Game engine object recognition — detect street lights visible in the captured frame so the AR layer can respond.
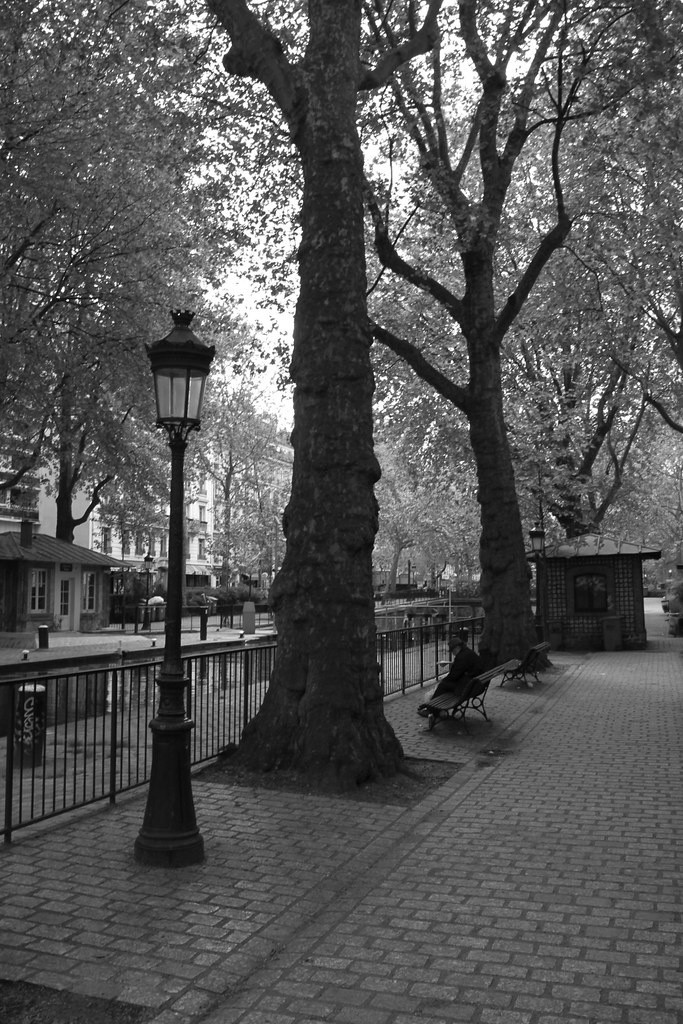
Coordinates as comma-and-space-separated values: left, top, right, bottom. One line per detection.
412, 562, 416, 584
142, 551, 154, 630
132, 304, 218, 870
528, 519, 544, 643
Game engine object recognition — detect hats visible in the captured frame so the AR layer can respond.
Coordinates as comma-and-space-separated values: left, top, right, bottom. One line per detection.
454, 626, 470, 642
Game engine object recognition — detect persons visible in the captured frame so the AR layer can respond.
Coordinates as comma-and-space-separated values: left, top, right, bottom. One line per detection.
416, 637, 482, 718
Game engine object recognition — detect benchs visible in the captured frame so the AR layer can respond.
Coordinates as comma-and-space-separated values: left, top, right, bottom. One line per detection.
495, 639, 553, 690
415, 656, 524, 737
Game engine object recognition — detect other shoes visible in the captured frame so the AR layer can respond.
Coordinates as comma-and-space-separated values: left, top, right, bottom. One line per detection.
419, 708, 431, 717
418, 702, 429, 710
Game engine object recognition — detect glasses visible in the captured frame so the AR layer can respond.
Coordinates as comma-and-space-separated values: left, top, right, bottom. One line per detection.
450, 635, 458, 639
